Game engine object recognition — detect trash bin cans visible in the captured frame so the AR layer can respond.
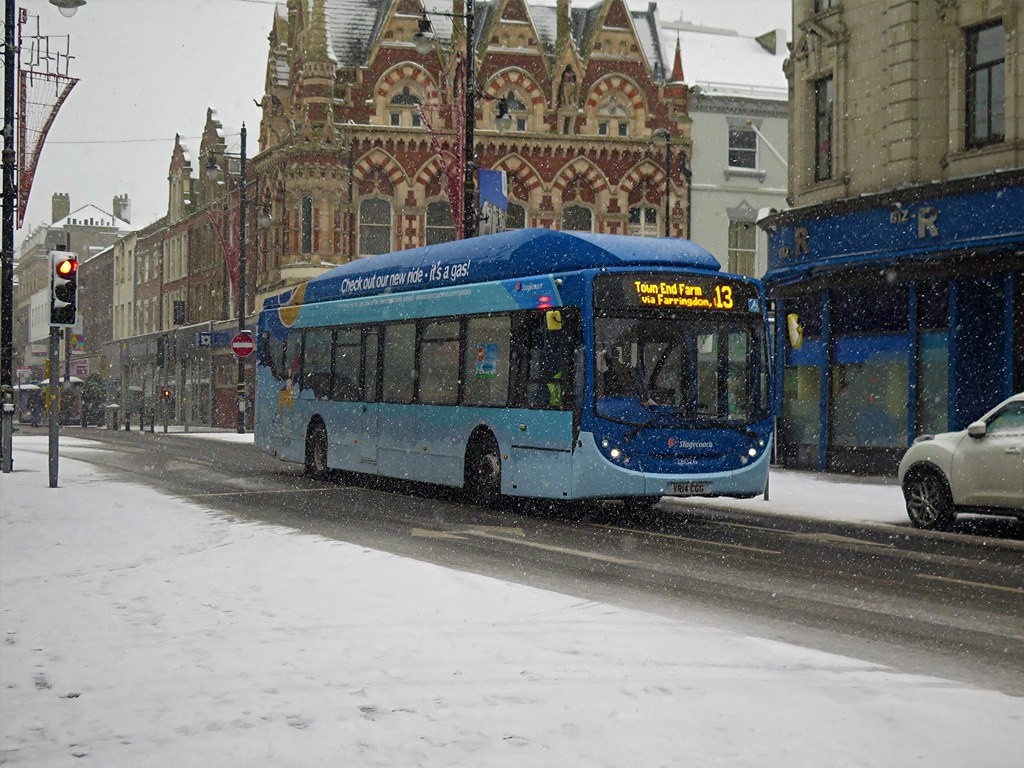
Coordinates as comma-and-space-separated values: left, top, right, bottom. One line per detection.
107, 404, 121, 430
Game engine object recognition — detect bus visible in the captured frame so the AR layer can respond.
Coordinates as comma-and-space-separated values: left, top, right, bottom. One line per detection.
253, 226, 805, 515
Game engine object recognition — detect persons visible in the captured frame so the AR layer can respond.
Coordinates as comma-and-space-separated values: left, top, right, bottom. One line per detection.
605, 346, 642, 397
29, 398, 41, 427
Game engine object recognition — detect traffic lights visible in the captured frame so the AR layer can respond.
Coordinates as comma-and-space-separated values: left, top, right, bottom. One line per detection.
48, 251, 79, 328
164, 391, 171, 396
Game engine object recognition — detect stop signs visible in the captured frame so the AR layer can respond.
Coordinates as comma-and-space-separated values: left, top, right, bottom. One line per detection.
231, 333, 254, 357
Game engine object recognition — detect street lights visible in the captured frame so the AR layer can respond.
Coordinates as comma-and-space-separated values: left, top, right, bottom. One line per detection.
205, 120, 247, 434
1, 0, 87, 470
414, 1, 513, 240
652, 128, 671, 237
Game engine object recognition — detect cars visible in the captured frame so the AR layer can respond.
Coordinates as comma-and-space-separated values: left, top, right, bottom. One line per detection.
897, 392, 1024, 532
